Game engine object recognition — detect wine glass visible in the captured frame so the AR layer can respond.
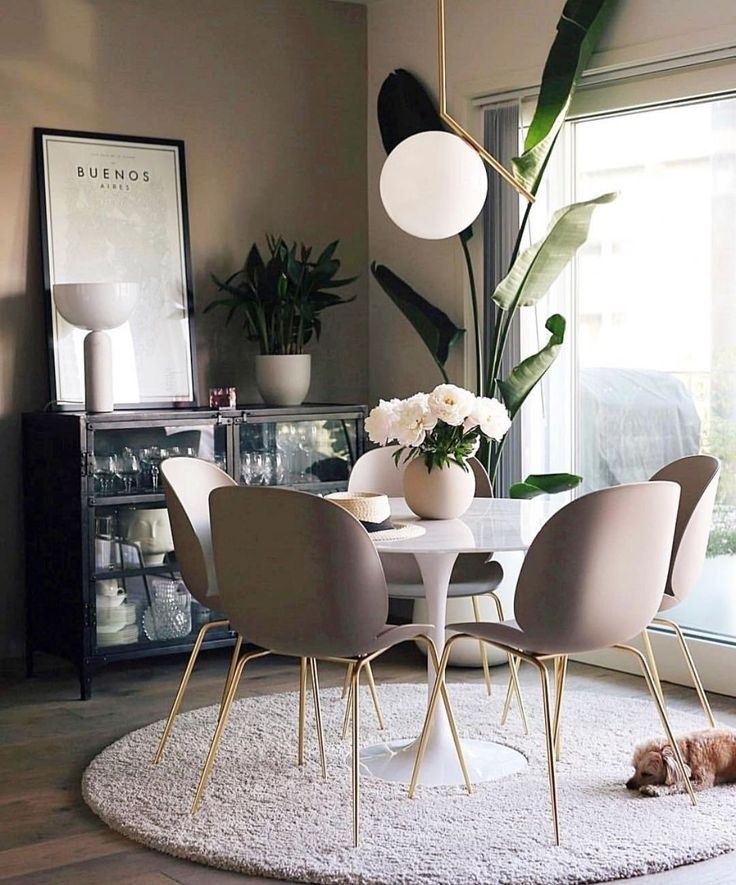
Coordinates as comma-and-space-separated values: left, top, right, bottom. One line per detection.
94, 446, 169, 496
276, 422, 322, 484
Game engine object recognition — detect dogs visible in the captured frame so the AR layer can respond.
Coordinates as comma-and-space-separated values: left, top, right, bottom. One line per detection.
625, 727, 736, 797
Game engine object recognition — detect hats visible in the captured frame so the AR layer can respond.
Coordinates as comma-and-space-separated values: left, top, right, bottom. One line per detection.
324, 491, 426, 541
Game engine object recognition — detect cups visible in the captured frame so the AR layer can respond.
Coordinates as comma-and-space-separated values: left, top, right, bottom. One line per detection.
96, 578, 125, 596
166, 447, 196, 457
241, 449, 290, 486
209, 387, 236, 410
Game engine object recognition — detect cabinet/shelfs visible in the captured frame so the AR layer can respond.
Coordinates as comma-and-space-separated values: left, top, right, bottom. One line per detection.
20, 407, 367, 698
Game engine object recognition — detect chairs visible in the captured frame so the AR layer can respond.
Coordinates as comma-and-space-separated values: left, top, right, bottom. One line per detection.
156, 445, 722, 850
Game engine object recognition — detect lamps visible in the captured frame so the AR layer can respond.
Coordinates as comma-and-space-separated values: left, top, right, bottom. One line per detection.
53, 280, 145, 414
380, 129, 488, 392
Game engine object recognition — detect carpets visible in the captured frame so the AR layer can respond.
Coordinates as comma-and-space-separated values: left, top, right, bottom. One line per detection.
84, 683, 736, 885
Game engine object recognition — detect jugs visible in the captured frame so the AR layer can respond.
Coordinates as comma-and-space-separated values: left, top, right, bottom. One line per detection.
142, 578, 193, 641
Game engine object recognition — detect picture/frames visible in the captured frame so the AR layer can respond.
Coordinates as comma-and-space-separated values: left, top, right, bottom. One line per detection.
34, 126, 198, 411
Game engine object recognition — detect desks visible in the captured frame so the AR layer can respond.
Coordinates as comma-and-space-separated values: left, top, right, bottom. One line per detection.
347, 497, 546, 787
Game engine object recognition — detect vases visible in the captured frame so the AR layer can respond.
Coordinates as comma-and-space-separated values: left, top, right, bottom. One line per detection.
404, 454, 476, 519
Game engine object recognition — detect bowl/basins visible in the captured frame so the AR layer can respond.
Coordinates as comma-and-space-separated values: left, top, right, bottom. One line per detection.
96, 594, 139, 648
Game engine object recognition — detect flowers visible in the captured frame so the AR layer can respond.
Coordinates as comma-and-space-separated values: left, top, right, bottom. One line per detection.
365, 382, 514, 474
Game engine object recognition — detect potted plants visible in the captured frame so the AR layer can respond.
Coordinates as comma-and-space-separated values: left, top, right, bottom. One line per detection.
203, 234, 358, 408
368, 0, 617, 668
